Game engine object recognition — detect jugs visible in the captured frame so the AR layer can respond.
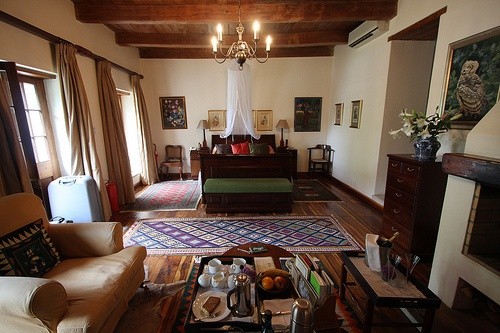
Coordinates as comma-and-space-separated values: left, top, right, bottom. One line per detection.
226, 272, 254, 318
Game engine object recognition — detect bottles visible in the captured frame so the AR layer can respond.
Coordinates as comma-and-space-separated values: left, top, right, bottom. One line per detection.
380, 232, 399, 247
261, 310, 274, 333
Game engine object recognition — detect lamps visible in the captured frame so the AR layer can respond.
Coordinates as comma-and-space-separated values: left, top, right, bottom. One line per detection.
210, 0, 274, 72
196, 119, 211, 153
275, 119, 290, 150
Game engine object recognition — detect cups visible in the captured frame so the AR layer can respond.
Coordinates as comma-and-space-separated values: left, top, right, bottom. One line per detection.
198, 271, 236, 289
230, 258, 246, 273
204, 260, 222, 274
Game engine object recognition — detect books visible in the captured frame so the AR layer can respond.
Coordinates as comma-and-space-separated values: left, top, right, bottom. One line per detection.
288, 253, 335, 308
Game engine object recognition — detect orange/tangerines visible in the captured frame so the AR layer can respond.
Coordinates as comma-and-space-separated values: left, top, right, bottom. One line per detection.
262, 276, 274, 289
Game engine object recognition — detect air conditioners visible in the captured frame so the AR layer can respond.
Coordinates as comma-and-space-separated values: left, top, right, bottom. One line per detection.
347, 20, 391, 50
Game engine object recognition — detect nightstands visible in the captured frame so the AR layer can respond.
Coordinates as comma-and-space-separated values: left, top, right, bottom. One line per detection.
276, 148, 298, 176
190, 152, 211, 180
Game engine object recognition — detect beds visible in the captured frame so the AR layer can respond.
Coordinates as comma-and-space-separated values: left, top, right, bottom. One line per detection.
197, 133, 294, 205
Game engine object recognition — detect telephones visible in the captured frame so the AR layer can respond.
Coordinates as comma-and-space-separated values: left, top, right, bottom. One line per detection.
190, 147, 196, 150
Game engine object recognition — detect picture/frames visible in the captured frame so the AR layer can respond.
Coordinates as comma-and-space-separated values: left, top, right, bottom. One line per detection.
294, 96, 322, 132
159, 96, 188, 130
349, 100, 363, 129
252, 110, 273, 131
333, 102, 345, 126
437, 25, 500, 131
208, 110, 225, 131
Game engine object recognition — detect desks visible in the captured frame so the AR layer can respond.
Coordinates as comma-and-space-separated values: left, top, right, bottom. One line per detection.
338, 249, 442, 333
181, 240, 297, 333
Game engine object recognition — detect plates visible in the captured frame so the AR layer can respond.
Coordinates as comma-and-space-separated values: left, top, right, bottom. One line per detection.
192, 290, 232, 322
204, 264, 227, 275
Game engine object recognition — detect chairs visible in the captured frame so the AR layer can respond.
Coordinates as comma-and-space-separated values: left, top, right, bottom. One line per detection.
0, 190, 149, 333
307, 144, 334, 179
158, 144, 184, 181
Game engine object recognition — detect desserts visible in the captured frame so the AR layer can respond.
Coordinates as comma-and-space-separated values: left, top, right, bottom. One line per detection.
201, 297, 220, 315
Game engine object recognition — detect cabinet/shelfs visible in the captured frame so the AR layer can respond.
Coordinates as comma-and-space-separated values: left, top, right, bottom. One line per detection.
380, 153, 449, 286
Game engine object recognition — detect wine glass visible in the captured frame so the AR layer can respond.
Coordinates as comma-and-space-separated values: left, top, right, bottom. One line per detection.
404, 254, 420, 289
384, 253, 402, 287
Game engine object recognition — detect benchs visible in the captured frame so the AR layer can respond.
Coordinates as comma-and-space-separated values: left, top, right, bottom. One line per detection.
203, 177, 293, 214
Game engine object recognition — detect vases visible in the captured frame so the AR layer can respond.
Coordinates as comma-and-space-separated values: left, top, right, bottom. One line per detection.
413, 136, 442, 159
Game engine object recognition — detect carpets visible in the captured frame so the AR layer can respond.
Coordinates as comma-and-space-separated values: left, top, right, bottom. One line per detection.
119, 179, 202, 214
172, 255, 369, 333
122, 213, 365, 254
291, 178, 344, 204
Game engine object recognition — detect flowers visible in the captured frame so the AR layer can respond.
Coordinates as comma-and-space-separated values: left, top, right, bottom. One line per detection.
388, 104, 463, 142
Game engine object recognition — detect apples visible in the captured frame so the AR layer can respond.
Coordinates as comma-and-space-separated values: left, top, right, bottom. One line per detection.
274, 276, 286, 289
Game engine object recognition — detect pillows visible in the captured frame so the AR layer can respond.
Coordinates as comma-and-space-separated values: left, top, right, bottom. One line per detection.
247, 141, 269, 155
212, 143, 233, 155
230, 141, 250, 155
0, 217, 63, 279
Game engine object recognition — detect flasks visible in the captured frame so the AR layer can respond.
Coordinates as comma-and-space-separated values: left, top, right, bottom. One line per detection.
290, 298, 313, 333
